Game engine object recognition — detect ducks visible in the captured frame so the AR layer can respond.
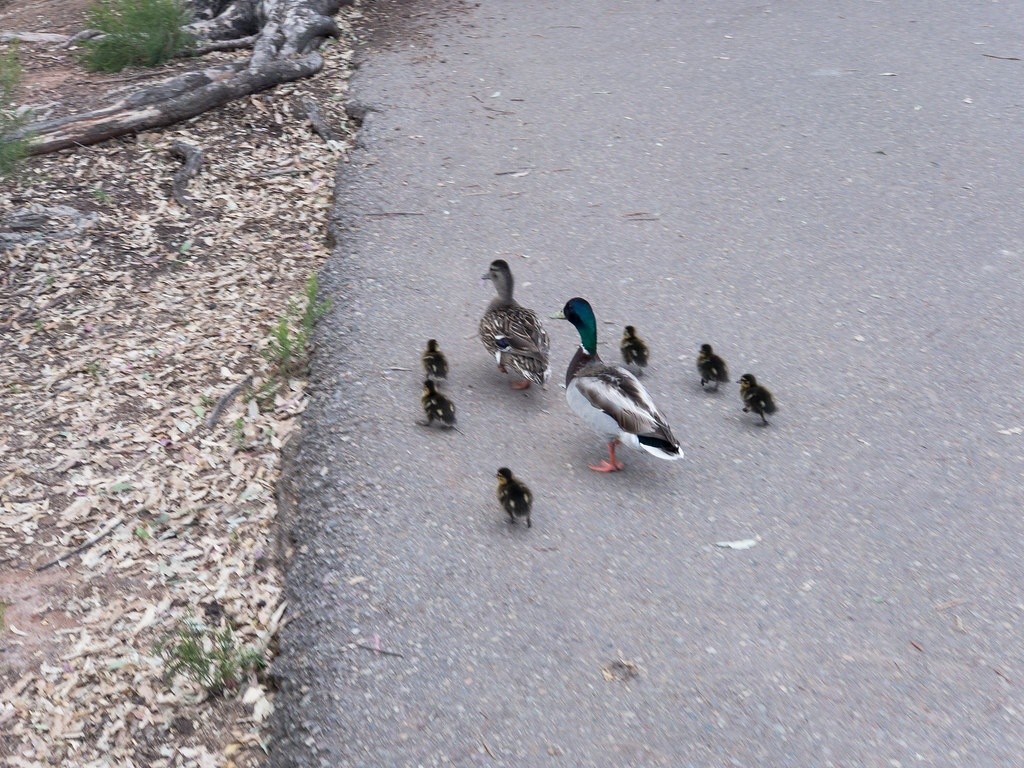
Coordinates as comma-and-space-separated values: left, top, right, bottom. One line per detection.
414, 378, 458, 428
495, 467, 534, 529
696, 343, 730, 394
548, 297, 685, 473
422, 338, 449, 386
477, 258, 551, 392
618, 325, 648, 373
736, 373, 776, 427
